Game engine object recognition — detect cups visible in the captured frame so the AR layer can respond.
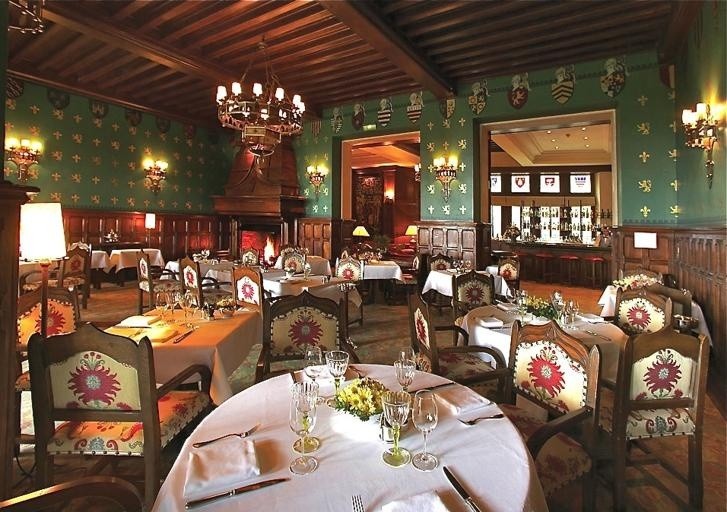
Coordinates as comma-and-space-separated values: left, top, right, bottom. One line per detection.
399, 345, 415, 359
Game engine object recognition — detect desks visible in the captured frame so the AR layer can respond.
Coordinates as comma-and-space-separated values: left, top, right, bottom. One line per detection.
305, 256, 329, 276
149, 364, 532, 510
101, 307, 263, 406
336, 258, 399, 305
90, 249, 161, 290
17, 257, 58, 295
159, 260, 365, 330
262, 272, 350, 317
600, 286, 700, 333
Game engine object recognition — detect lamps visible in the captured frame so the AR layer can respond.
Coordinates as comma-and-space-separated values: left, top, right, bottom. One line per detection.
145, 212, 156, 248
16, 203, 76, 336
432, 155, 460, 201
213, 34, 305, 169
141, 151, 169, 197
403, 225, 420, 244
304, 161, 328, 194
414, 163, 421, 182
681, 101, 724, 188
3, 138, 42, 183
352, 223, 369, 245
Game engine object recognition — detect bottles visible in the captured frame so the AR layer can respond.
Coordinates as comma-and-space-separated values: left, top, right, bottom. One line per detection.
521, 205, 610, 244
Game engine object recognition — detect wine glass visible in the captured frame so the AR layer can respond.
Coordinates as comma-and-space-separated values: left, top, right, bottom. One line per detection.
291, 381, 319, 474
155, 289, 199, 325
393, 358, 416, 388
451, 259, 472, 272
412, 388, 439, 470
323, 351, 348, 409
506, 285, 529, 326
303, 345, 324, 407
381, 388, 410, 468
551, 288, 580, 330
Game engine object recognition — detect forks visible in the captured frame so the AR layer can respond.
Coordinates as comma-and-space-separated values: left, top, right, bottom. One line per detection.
350, 364, 365, 379
587, 320, 610, 324
191, 423, 261, 449
456, 413, 505, 427
350, 494, 365, 512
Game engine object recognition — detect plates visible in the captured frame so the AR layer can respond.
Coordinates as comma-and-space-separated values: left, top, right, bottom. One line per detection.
475, 314, 503, 328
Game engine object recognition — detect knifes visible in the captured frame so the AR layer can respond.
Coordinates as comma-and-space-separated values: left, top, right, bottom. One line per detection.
184, 475, 292, 509
408, 382, 455, 394
489, 326, 509, 330
582, 329, 612, 342
442, 465, 484, 512
174, 329, 194, 344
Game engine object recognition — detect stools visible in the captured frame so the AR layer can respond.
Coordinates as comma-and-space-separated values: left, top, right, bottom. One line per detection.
514, 251, 608, 289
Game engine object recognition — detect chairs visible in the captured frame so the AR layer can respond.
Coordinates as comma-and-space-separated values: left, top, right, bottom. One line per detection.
598, 325, 709, 507
177, 255, 232, 309
496, 257, 519, 293
10, 286, 81, 459
28, 321, 212, 509
334, 256, 365, 330
452, 318, 603, 511
617, 269, 662, 284
452, 269, 511, 346
337, 244, 423, 306
406, 290, 507, 405
240, 243, 308, 274
230, 267, 263, 311
255, 289, 360, 383
136, 250, 180, 314
20, 241, 92, 309
418, 255, 456, 323
598, 284, 673, 337
642, 281, 692, 329
